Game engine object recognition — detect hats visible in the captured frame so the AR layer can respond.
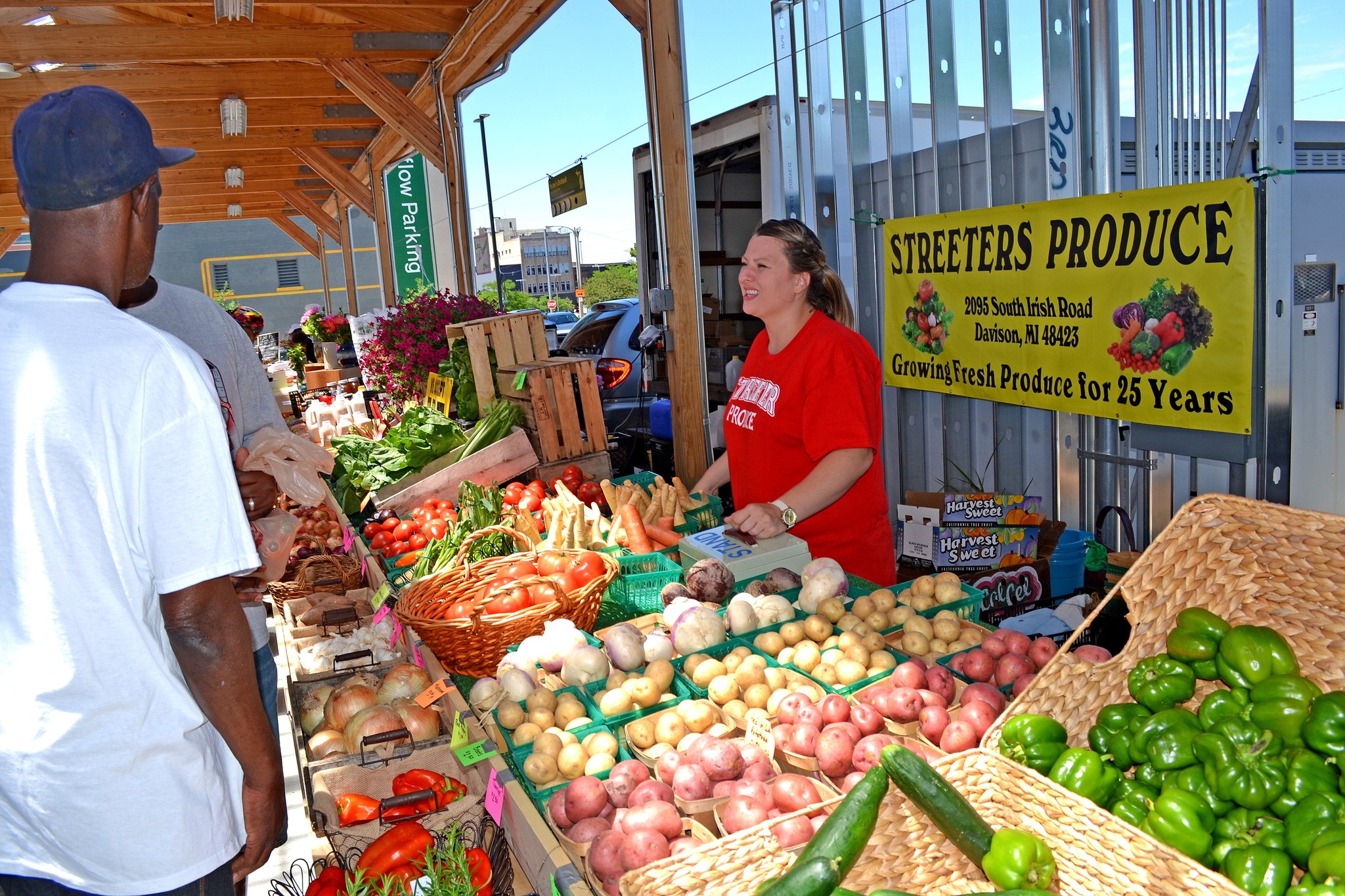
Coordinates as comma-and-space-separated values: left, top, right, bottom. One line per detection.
227, 306, 264, 331
12, 85, 198, 211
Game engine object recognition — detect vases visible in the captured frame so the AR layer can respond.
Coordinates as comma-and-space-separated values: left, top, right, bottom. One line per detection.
313, 341, 324, 363
338, 343, 356, 369
322, 342, 338, 370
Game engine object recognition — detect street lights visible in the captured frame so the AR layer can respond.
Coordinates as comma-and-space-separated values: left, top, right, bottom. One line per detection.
544, 225, 584, 319
473, 113, 503, 314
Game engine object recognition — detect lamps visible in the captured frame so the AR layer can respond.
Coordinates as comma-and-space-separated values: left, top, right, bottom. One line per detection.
227, 204, 242, 217
214, 0, 254, 25
220, 95, 247, 139
0, 63, 21, 79
225, 166, 243, 189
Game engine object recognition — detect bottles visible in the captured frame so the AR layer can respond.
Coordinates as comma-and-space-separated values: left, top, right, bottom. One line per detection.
701, 279, 704, 294
726, 356, 745, 392
301, 376, 374, 447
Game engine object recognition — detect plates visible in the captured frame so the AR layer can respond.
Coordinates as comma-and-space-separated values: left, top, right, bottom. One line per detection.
702, 294, 713, 297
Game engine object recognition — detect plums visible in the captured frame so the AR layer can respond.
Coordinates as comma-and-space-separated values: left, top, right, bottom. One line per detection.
358, 508, 400, 534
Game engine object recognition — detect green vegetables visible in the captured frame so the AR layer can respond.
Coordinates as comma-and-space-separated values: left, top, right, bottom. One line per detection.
900, 290, 955, 354
1137, 278, 1214, 351
325, 341, 527, 511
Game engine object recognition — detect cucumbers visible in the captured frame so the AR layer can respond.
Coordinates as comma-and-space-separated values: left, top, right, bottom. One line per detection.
931, 339, 941, 353
752, 745, 1065, 896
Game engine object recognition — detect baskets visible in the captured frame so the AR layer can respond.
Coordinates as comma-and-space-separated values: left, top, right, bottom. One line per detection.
288, 386, 337, 419
979, 491, 1345, 896
267, 535, 363, 623
619, 747, 1251, 896
355, 471, 723, 596
981, 587, 1106, 653
393, 525, 620, 679
490, 571, 1040, 896
608, 552, 683, 614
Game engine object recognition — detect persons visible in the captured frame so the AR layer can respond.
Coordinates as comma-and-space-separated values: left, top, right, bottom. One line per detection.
0, 85, 288, 896
118, 276, 290, 741
689, 218, 897, 588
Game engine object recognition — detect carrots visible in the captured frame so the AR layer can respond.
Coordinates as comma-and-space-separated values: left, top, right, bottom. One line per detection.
617, 503, 689, 570
1120, 319, 1141, 343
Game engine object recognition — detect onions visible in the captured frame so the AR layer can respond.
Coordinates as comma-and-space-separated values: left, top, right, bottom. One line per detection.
299, 661, 441, 760
282, 500, 348, 562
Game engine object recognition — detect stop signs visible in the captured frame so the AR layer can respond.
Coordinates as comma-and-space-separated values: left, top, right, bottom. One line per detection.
548, 300, 557, 309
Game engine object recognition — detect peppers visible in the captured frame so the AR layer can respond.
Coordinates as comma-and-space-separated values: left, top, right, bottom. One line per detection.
1153, 312, 1185, 350
303, 768, 494, 896
981, 608, 1345, 896
394, 552, 422, 567
1160, 342, 1193, 376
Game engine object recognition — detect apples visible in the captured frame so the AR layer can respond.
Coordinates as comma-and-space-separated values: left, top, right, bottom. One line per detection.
917, 279, 933, 333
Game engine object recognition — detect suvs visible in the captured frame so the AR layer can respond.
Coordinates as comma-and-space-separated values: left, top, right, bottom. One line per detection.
506, 308, 579, 343
556, 296, 658, 443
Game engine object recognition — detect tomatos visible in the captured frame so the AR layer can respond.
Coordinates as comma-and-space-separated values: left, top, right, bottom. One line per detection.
456, 465, 611, 529
364, 498, 469, 557
1106, 342, 1163, 374
444, 549, 607, 620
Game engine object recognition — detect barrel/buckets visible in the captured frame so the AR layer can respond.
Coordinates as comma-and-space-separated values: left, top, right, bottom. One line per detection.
1049, 530, 1096, 604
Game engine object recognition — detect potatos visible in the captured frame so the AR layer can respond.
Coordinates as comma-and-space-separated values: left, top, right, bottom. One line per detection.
754, 571, 1113, 755
292, 566, 376, 627
494, 644, 930, 896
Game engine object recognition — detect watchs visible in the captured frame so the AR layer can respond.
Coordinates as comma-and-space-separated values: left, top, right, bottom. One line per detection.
771, 499, 797, 530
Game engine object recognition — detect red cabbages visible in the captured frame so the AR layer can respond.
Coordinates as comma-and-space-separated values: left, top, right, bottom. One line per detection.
1112, 302, 1145, 330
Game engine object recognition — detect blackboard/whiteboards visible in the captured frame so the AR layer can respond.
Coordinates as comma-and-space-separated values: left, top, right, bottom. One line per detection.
257, 331, 280, 360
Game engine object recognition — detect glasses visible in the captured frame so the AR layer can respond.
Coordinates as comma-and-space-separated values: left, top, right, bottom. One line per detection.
248, 327, 261, 338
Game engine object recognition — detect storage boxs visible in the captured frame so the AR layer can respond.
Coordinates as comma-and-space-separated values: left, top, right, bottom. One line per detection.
896, 489, 1143, 657
700, 293, 755, 381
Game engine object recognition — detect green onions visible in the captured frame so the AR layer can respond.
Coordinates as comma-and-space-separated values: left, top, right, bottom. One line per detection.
399, 478, 513, 595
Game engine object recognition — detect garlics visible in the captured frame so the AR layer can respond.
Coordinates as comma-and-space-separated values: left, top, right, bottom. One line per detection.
298, 614, 398, 673
928, 312, 936, 327
1144, 318, 1160, 333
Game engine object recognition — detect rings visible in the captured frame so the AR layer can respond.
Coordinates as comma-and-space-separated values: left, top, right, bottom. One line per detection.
248, 497, 256, 512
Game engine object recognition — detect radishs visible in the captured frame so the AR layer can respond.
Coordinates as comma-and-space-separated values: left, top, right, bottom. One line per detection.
664, 556, 850, 656
513, 476, 719, 554
466, 617, 672, 704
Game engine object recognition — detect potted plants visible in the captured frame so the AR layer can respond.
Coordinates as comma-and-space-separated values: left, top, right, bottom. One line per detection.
284, 341, 307, 391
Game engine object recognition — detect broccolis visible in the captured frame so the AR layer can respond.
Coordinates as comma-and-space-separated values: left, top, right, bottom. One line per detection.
1129, 330, 1161, 359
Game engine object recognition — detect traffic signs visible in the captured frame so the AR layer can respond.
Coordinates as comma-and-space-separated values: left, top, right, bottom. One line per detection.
575, 288, 585, 298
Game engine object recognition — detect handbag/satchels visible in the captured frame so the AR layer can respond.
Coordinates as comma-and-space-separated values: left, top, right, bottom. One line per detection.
1083, 506, 1142, 591
232, 427, 335, 591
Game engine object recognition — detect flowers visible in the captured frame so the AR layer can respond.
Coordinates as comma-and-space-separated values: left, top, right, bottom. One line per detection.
301, 304, 354, 345
356, 290, 510, 408
